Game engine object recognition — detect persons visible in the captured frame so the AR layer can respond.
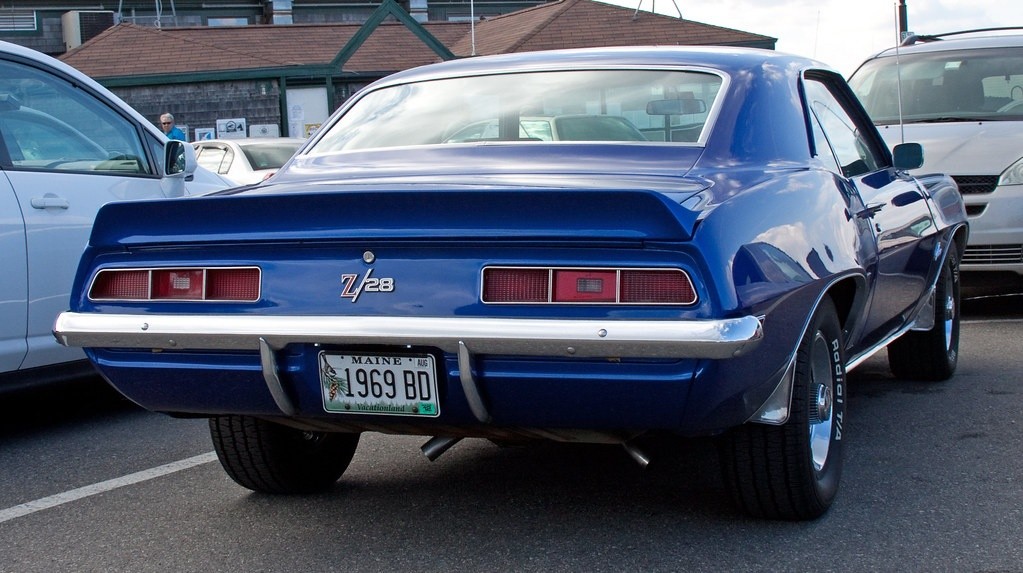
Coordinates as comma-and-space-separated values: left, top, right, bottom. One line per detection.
160, 113, 185, 142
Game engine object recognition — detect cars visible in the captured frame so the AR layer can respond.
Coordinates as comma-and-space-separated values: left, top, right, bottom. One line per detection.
184, 136, 309, 187
845, 25, 1023, 316
0, 40, 242, 394
51, 45, 972, 519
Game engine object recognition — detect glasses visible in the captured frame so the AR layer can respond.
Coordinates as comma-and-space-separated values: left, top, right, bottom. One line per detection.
162, 121, 172, 125
